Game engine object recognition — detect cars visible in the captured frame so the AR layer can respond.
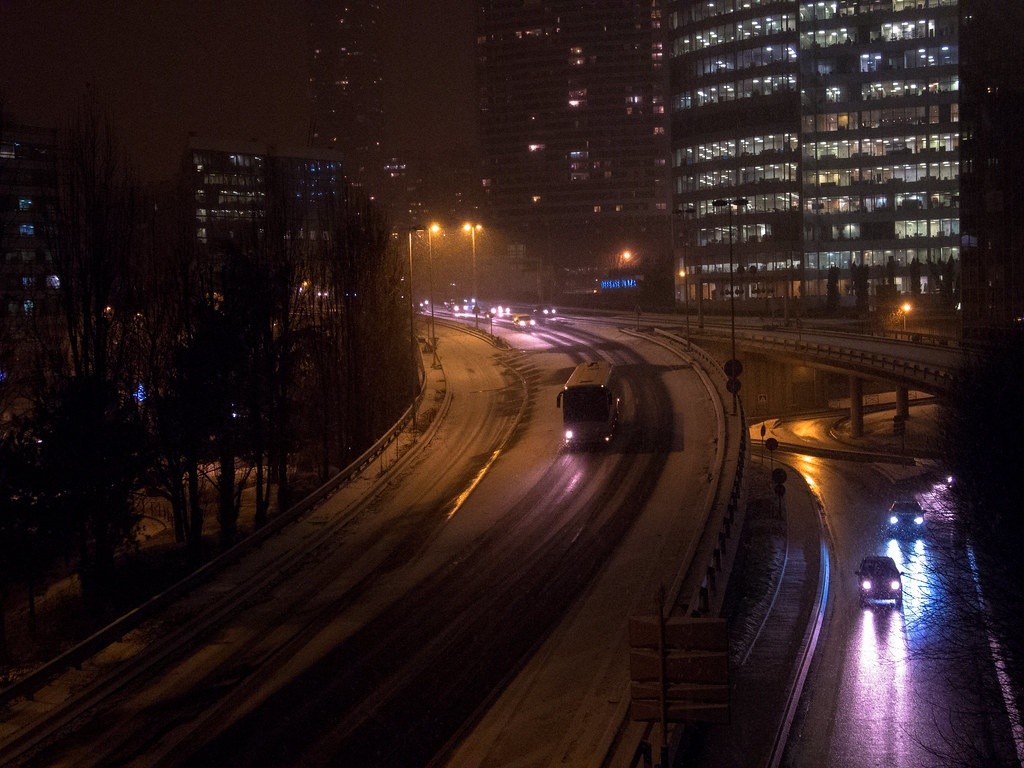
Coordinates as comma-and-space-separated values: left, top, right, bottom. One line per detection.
533, 304, 556, 318
854, 554, 906, 608
886, 499, 928, 533
418, 296, 511, 317
514, 313, 535, 332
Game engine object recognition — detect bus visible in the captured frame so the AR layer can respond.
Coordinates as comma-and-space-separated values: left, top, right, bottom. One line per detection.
555, 359, 624, 450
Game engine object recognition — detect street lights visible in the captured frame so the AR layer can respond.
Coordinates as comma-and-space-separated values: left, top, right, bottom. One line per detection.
901, 302, 910, 332
620, 249, 640, 331
463, 221, 484, 330
711, 195, 750, 414
426, 223, 441, 371
670, 208, 700, 353
407, 225, 424, 429
